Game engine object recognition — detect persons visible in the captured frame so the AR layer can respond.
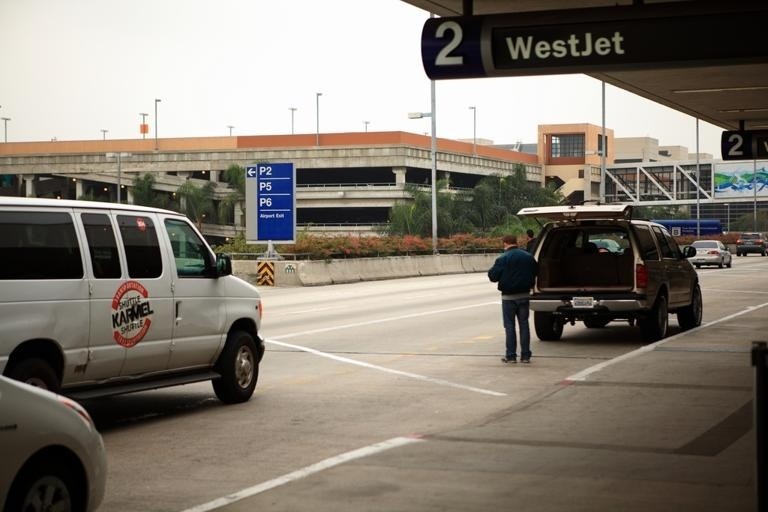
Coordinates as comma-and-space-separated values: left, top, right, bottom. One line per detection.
486, 235, 537, 364
525, 228, 539, 255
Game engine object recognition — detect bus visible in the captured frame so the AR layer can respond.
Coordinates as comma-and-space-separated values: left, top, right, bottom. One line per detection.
648, 218, 721, 238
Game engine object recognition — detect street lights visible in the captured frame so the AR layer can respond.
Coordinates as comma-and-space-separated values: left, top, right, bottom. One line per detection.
723, 203, 729, 233
140, 113, 148, 139
288, 107, 297, 134
407, 80, 437, 254
0, 117, 11, 143
154, 98, 162, 149
227, 126, 234, 135
316, 93, 323, 146
467, 105, 476, 154
100, 130, 108, 140
365, 121, 369, 133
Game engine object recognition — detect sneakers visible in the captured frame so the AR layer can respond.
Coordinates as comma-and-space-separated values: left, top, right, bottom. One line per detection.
521, 359, 530, 363
502, 357, 517, 363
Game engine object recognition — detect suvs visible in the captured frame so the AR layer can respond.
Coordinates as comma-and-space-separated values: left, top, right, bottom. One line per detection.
513, 201, 703, 343
735, 232, 767, 258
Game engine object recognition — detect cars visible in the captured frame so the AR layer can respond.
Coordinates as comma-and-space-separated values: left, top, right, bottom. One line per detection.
686, 240, 731, 268
1, 373, 108, 512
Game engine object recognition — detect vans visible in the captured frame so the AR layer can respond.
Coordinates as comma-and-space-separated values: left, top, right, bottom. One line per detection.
0, 195, 266, 409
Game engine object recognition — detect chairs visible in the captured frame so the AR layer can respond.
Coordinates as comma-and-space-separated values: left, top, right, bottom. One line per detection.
580, 242, 598, 255
